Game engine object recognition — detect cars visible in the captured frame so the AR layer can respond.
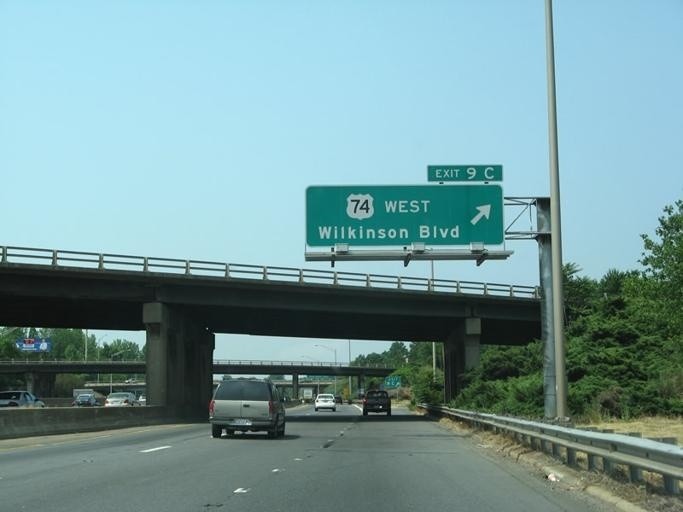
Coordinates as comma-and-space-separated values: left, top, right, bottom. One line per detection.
314, 393, 342, 412
0, 378, 146, 407
208, 377, 285, 439
363, 390, 391, 416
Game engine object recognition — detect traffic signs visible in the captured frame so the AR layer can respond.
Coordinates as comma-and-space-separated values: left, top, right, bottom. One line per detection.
384, 375, 401, 388
305, 164, 504, 245
